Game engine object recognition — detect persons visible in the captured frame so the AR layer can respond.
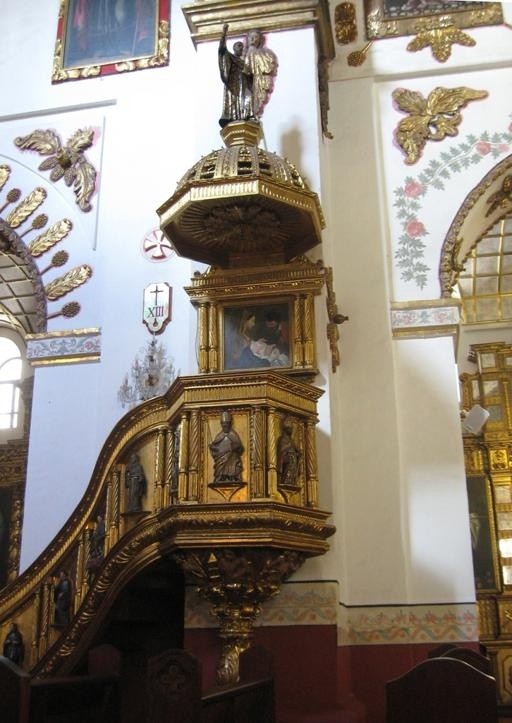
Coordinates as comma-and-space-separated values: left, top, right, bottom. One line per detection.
3, 623, 24, 662
241, 28, 276, 115
207, 412, 244, 482
125, 454, 146, 510
92, 516, 105, 555
218, 23, 257, 128
276, 418, 303, 485
53, 570, 72, 626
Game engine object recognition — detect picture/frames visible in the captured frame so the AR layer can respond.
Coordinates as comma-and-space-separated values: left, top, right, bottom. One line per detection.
348, 0, 512, 67
217, 295, 295, 375
51, 0, 172, 85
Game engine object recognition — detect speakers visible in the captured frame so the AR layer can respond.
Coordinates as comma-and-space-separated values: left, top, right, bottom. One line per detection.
462, 403, 489, 436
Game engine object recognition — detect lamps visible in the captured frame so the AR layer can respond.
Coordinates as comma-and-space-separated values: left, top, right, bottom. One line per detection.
459, 405, 490, 435
